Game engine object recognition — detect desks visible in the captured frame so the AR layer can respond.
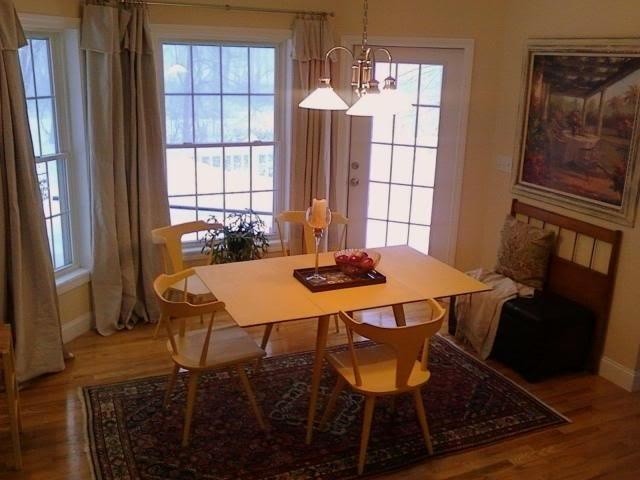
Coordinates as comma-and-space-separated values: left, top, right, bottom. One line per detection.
192, 242, 495, 444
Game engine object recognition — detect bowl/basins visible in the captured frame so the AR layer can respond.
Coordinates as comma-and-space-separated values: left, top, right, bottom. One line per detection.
333, 249, 382, 275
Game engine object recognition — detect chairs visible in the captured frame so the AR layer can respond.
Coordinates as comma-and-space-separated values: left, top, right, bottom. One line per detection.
150, 218, 225, 342
256, 208, 354, 356
148, 266, 272, 447
315, 295, 447, 476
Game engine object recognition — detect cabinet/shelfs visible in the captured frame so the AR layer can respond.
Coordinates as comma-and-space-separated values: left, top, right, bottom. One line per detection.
1, 324, 25, 472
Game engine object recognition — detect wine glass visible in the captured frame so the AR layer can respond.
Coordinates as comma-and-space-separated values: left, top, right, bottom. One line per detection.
305, 205, 331, 282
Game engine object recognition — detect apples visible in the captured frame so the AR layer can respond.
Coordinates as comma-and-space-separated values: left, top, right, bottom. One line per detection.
335, 251, 373, 275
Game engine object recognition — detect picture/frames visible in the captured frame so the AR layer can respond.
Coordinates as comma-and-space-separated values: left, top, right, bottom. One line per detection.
508, 32, 639, 228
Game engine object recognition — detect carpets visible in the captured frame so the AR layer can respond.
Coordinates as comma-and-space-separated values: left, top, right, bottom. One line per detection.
73, 326, 575, 479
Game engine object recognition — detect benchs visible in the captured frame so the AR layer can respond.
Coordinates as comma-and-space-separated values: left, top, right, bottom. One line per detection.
447, 197, 624, 384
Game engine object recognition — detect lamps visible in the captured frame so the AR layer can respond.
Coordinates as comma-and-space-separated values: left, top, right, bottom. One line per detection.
296, 0, 418, 119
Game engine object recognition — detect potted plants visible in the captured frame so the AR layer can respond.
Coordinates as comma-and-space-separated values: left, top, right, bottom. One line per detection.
200, 208, 270, 264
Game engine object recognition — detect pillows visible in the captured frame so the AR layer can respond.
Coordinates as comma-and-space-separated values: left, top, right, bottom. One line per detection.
494, 215, 558, 295
